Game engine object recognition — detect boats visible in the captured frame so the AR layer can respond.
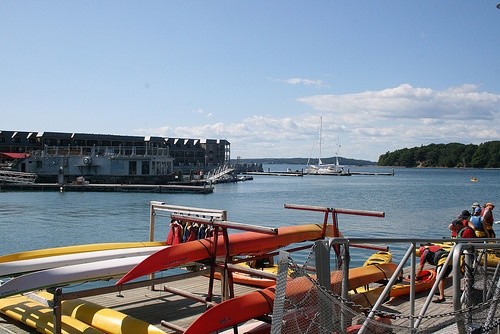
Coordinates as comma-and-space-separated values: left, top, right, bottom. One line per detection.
305, 114, 347, 174
390, 268, 438, 297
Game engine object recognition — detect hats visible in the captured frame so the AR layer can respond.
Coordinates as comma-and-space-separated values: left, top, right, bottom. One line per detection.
486, 203, 495, 207
472, 202, 481, 208
462, 210, 471, 217
474, 207, 481, 214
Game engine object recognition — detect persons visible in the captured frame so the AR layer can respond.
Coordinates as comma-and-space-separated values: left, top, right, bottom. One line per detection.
449, 202, 496, 253
416, 243, 452, 303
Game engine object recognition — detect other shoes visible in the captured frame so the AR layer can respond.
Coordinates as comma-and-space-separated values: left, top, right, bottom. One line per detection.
432, 298, 446, 303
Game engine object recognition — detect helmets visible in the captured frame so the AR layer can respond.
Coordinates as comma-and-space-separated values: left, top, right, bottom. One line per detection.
416, 248, 420, 257
